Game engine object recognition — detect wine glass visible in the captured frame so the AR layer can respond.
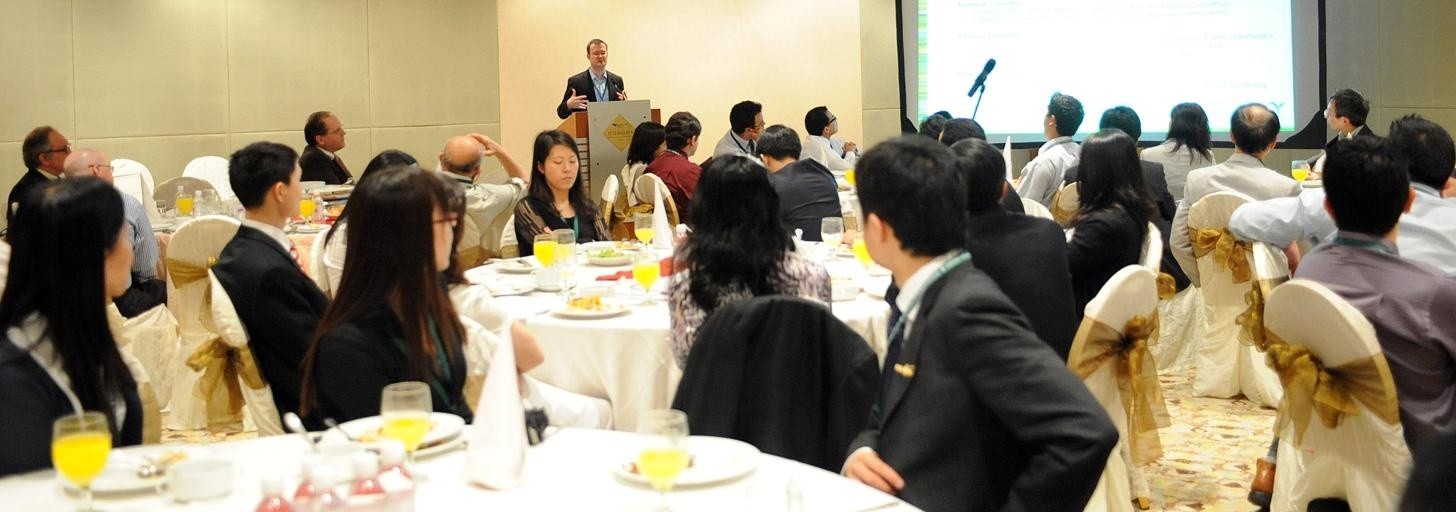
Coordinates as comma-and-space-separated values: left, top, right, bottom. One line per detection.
633, 213, 655, 245
846, 170, 858, 196
1290, 159, 1310, 184
532, 229, 577, 269
636, 407, 692, 492
380, 381, 434, 451
632, 251, 661, 306
820, 217, 845, 262
50, 411, 112, 511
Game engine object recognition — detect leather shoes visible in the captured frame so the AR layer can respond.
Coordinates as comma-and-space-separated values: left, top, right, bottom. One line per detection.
1248, 458, 1276, 506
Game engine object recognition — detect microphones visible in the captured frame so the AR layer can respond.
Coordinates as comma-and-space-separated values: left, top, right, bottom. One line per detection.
968, 58, 996, 98
610, 77, 627, 100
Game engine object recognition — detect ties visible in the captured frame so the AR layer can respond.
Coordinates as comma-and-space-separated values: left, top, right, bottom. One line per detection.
749, 139, 755, 155
884, 308, 904, 380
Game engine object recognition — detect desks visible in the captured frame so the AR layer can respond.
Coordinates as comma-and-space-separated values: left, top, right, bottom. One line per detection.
459, 240, 895, 434
0, 434, 923, 512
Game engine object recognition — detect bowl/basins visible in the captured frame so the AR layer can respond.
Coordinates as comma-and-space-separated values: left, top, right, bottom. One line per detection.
830, 170, 846, 192
791, 230, 893, 299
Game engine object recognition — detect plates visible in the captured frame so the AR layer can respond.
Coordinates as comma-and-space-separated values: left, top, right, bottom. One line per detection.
493, 279, 574, 297
412, 436, 468, 459
494, 259, 530, 272
556, 298, 626, 318
575, 238, 642, 265
618, 432, 763, 487
322, 410, 464, 457
89, 442, 188, 493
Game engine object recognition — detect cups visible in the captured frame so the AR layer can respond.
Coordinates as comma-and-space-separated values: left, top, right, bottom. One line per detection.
157, 184, 333, 233
158, 455, 231, 503
530, 267, 558, 286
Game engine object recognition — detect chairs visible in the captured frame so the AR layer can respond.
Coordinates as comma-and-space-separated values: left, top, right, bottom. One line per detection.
1192, 153, 1329, 407
1261, 277, 1412, 509
107, 155, 332, 446
1014, 175, 1164, 508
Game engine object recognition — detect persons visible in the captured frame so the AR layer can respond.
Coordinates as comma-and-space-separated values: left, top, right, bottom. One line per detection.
432, 132, 530, 275
299, 110, 355, 186
0, 174, 144, 480
513, 129, 613, 256
62, 148, 158, 290
619, 98, 1456, 511
323, 149, 420, 304
556, 38, 628, 120
299, 164, 474, 428
214, 141, 329, 435
4, 125, 73, 246
